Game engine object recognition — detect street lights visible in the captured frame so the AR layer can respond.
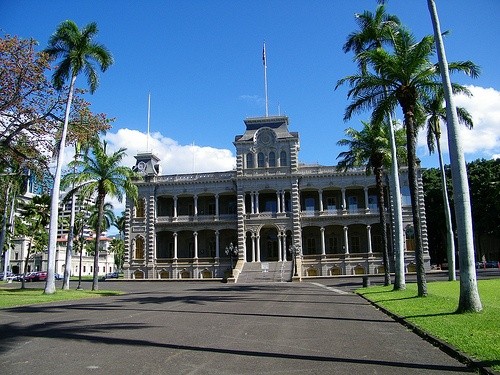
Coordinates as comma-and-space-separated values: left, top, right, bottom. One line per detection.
289, 244, 301, 275
224, 243, 238, 270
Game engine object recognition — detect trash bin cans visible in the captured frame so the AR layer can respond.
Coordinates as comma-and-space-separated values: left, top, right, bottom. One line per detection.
362, 276, 370, 288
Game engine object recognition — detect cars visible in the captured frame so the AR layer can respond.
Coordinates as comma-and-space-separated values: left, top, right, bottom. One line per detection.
102, 273, 118, 279
0, 270, 61, 282
475, 261, 500, 269
